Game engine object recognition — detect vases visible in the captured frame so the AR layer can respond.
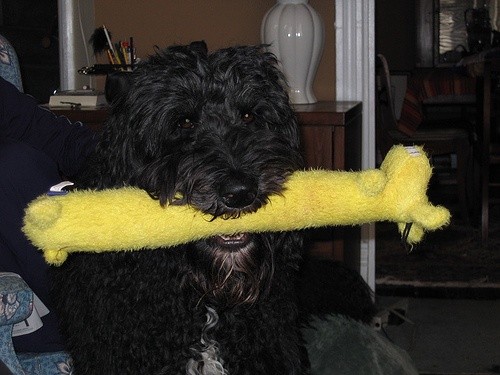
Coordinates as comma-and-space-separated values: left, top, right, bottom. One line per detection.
260, 0, 327, 105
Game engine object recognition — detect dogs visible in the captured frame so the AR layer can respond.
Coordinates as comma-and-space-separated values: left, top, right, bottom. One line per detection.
54, 39, 406, 374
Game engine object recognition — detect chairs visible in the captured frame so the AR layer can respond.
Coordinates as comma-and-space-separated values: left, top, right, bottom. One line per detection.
375, 54, 500, 242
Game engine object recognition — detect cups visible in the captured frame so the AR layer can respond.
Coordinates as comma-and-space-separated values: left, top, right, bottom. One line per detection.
103, 71, 136, 106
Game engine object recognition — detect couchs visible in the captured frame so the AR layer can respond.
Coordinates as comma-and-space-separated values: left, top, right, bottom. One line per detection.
0, 37, 77, 375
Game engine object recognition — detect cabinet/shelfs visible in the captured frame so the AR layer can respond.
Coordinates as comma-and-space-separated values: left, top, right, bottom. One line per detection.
40, 100, 364, 275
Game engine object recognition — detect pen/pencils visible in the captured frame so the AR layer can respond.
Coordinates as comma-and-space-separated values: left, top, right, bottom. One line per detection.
102, 23, 141, 72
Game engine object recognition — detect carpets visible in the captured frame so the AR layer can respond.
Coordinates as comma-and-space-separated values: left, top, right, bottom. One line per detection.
373, 215, 500, 301
